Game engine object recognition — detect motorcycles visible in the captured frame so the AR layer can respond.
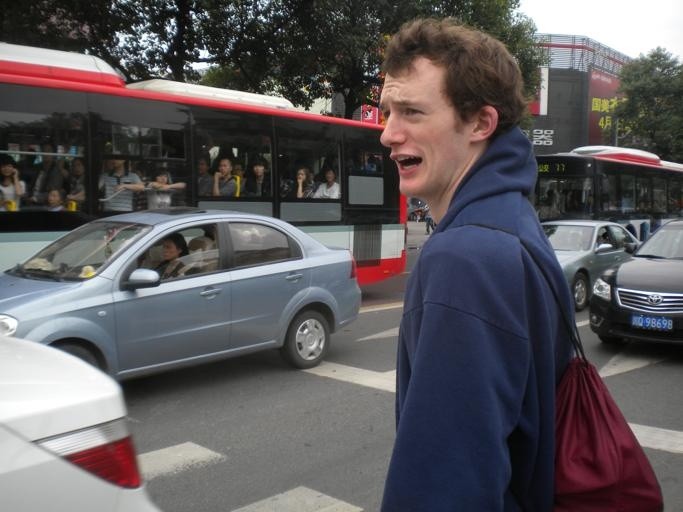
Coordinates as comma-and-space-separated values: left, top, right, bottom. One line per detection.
417, 200, 437, 232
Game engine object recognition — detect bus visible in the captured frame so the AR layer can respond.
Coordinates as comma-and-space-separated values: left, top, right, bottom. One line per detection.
531, 144, 682, 224
0, 42, 408, 292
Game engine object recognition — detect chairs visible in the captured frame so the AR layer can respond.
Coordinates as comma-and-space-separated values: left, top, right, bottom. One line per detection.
229, 174, 241, 199
185, 233, 218, 276
66, 199, 78, 212
0, 198, 17, 212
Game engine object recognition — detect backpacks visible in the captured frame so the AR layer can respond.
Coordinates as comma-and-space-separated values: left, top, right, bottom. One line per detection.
436, 222, 665, 512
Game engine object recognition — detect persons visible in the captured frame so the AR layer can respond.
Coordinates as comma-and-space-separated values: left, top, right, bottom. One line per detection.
374, 15, 580, 512
422, 203, 436, 235
154, 232, 201, 281
544, 178, 683, 219
2, 137, 386, 215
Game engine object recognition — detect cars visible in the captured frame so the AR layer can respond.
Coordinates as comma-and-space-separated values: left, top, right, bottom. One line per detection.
409, 201, 426, 222
0, 336, 157, 511
538, 220, 643, 312
0, 211, 361, 384
586, 220, 683, 354
